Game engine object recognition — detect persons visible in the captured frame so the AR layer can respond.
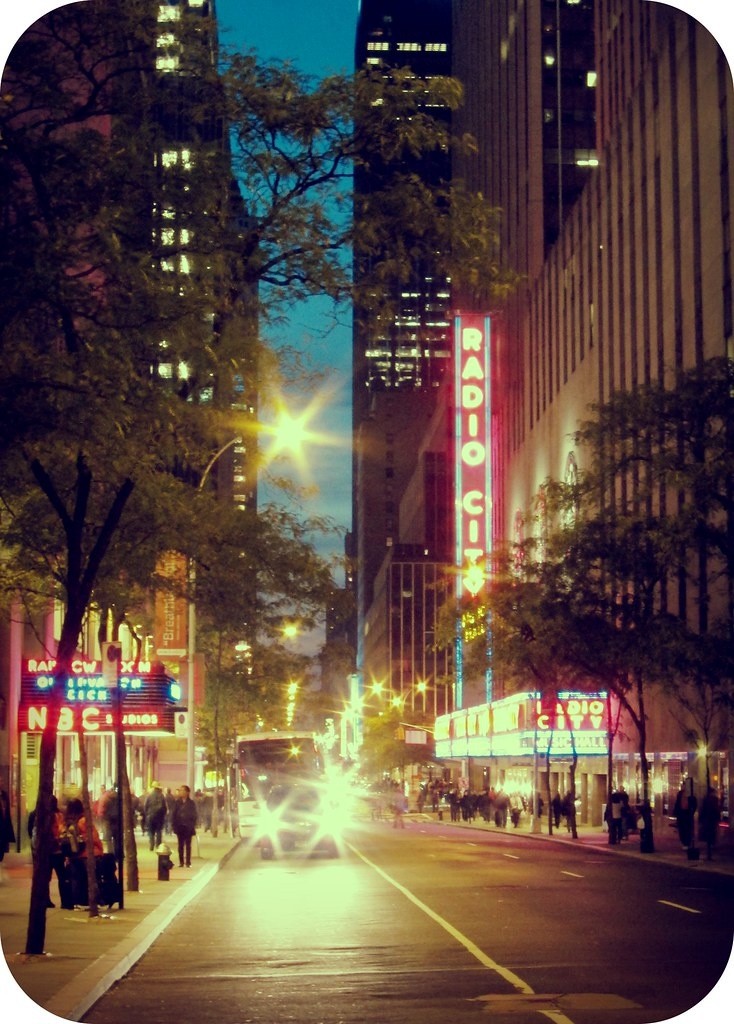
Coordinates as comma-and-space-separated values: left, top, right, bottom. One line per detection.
1, 760, 734, 913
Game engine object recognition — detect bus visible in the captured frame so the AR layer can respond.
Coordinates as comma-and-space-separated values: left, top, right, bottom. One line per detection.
232, 729, 336, 862
